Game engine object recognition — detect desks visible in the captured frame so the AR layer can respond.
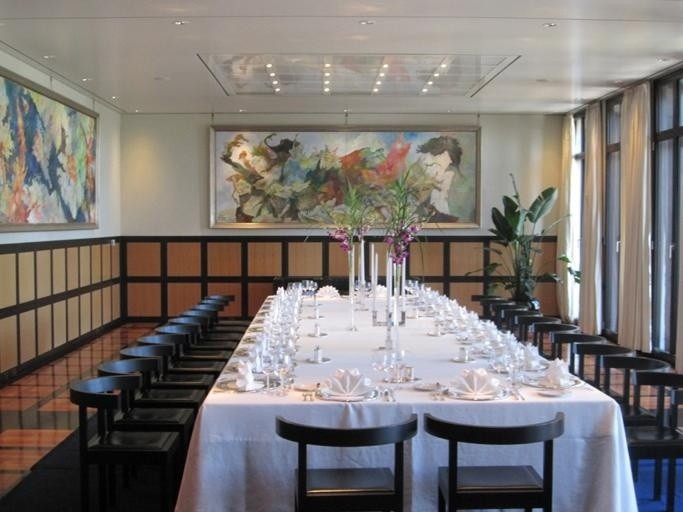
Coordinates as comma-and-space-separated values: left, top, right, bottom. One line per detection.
184, 295, 625, 512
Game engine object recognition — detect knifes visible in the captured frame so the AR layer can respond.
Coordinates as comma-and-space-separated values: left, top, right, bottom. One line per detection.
508, 388, 524, 400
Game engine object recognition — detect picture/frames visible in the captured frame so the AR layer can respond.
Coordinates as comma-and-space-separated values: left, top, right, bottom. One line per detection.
0, 65, 101, 233
209, 124, 481, 230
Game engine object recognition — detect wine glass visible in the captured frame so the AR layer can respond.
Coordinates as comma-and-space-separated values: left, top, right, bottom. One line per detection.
402, 279, 540, 391
372, 350, 403, 383
259, 279, 317, 397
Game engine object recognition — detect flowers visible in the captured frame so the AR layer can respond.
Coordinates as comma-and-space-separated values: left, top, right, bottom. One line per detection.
318, 165, 434, 295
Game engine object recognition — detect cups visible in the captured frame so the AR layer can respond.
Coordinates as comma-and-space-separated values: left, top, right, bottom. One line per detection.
379, 386, 394, 402
354, 280, 370, 299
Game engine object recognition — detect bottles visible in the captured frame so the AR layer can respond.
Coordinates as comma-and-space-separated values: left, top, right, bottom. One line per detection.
310, 294, 321, 362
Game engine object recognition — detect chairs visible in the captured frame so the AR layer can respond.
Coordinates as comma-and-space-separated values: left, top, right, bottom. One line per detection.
70, 295, 270, 509
425, 411, 566, 512
479, 294, 682, 506
270, 413, 417, 512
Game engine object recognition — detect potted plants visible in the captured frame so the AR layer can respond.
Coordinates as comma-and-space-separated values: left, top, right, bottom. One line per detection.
465, 174, 581, 311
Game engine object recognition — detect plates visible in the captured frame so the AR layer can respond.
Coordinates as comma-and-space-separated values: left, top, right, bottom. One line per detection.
520, 362, 548, 372
316, 382, 377, 402
526, 374, 582, 388
218, 317, 268, 392
306, 301, 330, 366
445, 385, 509, 401
